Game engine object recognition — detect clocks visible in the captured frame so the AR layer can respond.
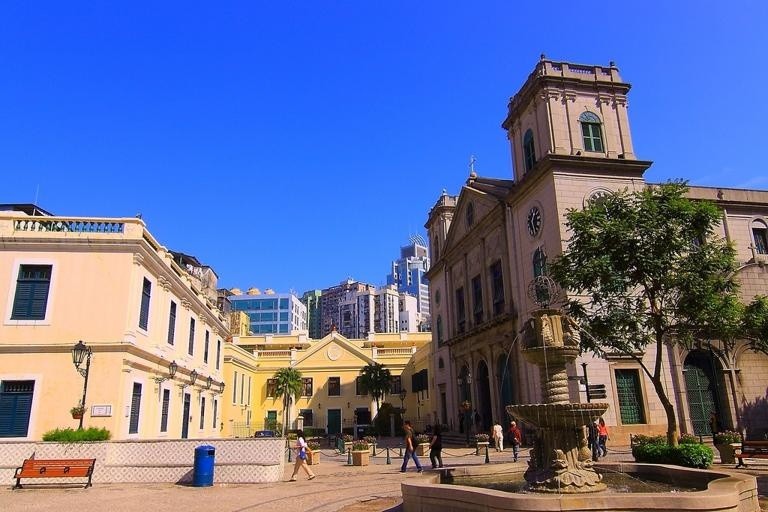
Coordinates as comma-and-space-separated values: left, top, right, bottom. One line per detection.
523, 199, 545, 240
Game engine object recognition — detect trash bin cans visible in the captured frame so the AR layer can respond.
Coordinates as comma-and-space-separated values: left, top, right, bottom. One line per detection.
192, 444, 216, 488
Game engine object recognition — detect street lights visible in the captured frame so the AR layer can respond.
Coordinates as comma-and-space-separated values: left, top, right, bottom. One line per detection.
398, 388, 408, 436
70, 340, 91, 430
706, 322, 738, 432
457, 365, 475, 447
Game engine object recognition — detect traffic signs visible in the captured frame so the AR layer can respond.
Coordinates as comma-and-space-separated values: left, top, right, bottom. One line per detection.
589, 395, 606, 399
567, 376, 585, 380
580, 380, 587, 385
588, 390, 606, 394
587, 384, 605, 389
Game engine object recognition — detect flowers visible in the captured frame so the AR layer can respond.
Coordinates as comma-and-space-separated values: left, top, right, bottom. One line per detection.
709, 431, 742, 445
308, 441, 320, 450
415, 433, 430, 442
338, 432, 376, 451
474, 432, 490, 441
70, 405, 86, 414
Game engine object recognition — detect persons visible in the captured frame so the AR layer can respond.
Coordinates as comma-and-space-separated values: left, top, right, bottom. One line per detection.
492, 420, 504, 452
288, 429, 316, 481
589, 422, 599, 462
457, 408, 465, 432
429, 423, 444, 470
708, 410, 718, 441
398, 419, 424, 473
597, 417, 611, 457
509, 420, 521, 462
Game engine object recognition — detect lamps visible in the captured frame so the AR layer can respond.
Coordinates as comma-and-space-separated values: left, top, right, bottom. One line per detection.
155, 359, 226, 397
455, 371, 473, 385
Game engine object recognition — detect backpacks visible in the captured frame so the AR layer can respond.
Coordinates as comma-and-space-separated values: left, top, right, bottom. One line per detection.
509, 429, 520, 445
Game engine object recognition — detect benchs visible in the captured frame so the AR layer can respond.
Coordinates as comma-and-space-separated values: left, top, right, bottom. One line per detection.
14, 458, 95, 489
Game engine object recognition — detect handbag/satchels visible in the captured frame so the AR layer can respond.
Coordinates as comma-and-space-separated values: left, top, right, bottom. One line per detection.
300, 450, 306, 459
411, 435, 419, 448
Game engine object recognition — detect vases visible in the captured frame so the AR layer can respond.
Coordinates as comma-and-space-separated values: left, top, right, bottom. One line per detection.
72, 413, 82, 419
712, 441, 738, 465
415, 444, 430, 454
342, 443, 373, 466
476, 442, 490, 455
304, 450, 320, 465
